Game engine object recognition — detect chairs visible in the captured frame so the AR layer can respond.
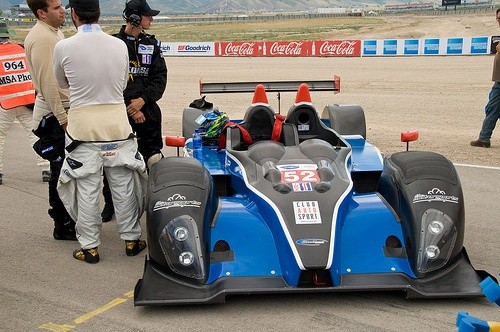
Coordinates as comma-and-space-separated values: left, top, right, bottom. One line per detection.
226, 102, 351, 150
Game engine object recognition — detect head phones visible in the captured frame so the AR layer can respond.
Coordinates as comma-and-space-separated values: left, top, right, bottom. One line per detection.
122, 12, 141, 27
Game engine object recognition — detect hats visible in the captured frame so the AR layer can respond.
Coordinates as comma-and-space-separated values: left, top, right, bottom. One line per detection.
65, 0, 100, 10
126, 0, 160, 17
0, 19, 16, 38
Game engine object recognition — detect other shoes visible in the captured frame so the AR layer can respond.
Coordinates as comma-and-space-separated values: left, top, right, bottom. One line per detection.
102, 205, 114, 222
124, 240, 146, 256
53, 227, 77, 240
42, 170, 51, 181
73, 248, 100, 264
471, 140, 490, 148
0, 174, 2, 184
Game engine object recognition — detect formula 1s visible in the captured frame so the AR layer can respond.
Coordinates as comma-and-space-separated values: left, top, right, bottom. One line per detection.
132, 74, 500, 307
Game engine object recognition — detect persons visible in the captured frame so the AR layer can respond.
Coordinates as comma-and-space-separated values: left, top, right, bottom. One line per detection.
101, 0, 168, 222
470, 8, 500, 147
53, 0, 149, 265
24, 0, 79, 240
